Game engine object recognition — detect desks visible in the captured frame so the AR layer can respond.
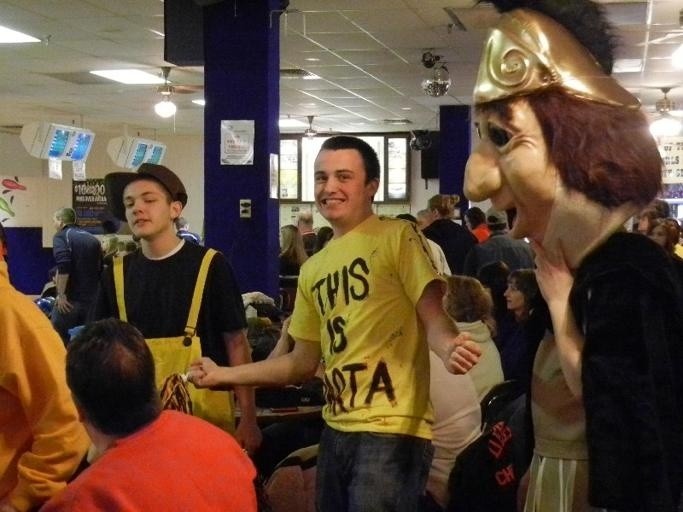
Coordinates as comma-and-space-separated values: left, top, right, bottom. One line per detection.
234, 405, 322, 426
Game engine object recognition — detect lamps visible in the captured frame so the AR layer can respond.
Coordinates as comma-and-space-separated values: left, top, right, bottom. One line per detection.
649, 85, 682, 137
154, 65, 176, 117
305, 116, 317, 140
421, 40, 451, 97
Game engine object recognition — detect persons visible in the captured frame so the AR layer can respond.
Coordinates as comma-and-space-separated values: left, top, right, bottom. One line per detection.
461, 1, 664, 272
296, 209, 316, 257
278, 224, 309, 276
631, 198, 669, 237
186, 136, 482, 512
0, 223, 93, 512
490, 269, 548, 381
91, 163, 264, 459
175, 216, 200, 245
645, 217, 682, 260
443, 273, 503, 403
38, 320, 254, 510
464, 206, 536, 290
530, 233, 682, 511
464, 208, 491, 243
48, 209, 104, 348
517, 310, 607, 512
422, 193, 480, 278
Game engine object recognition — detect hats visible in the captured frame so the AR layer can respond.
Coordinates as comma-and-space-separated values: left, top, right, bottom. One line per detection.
472, 0, 641, 111
105, 163, 187, 222
485, 206, 506, 225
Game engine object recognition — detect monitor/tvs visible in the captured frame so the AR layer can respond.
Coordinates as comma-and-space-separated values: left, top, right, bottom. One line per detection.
145, 139, 167, 165
65, 125, 95, 162
19, 121, 74, 160
106, 136, 149, 169
